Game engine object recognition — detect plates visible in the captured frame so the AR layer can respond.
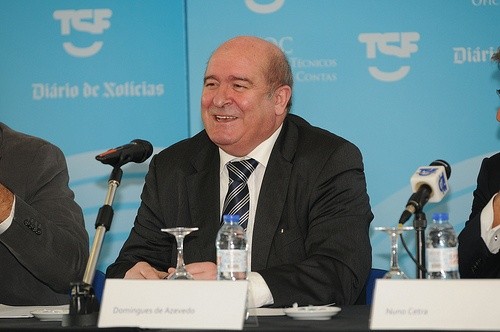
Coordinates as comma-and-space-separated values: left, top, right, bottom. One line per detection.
30, 310, 70, 321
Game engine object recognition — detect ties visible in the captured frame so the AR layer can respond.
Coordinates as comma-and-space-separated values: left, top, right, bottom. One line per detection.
220, 158, 260, 232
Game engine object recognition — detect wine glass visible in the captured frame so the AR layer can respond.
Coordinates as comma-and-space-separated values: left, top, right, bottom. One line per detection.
375, 227, 414, 279
160, 227, 199, 280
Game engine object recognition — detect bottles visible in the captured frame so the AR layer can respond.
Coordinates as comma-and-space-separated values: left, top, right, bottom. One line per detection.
425, 213, 460, 279
215, 215, 250, 319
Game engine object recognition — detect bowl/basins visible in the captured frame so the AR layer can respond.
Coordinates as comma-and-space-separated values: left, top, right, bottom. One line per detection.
283, 306, 342, 320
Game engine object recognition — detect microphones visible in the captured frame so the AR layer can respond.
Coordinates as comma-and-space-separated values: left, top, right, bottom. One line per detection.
398, 159, 451, 225
95, 139, 153, 167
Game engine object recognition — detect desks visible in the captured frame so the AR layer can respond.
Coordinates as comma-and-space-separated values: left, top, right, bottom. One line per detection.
0, 302, 500, 332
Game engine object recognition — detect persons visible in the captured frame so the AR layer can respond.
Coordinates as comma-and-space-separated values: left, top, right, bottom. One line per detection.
0, 122, 89, 306
105, 36, 374, 308
456, 48, 500, 279
